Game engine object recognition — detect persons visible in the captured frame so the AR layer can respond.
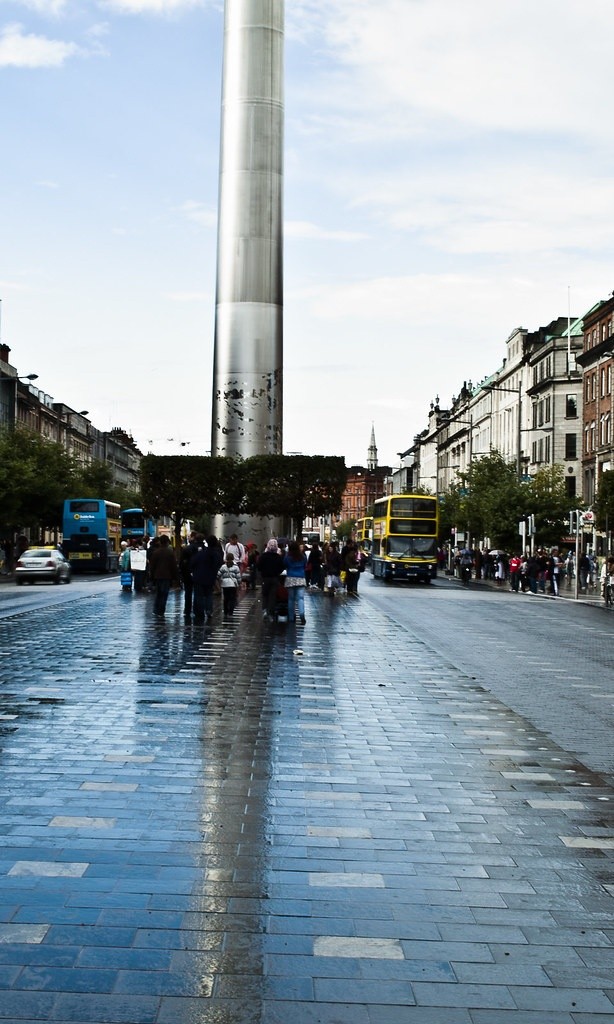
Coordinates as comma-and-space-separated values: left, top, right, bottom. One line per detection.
118, 531, 369, 624
437, 546, 614, 606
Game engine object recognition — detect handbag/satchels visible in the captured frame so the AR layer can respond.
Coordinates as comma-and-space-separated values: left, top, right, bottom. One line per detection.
121, 571, 132, 585
130, 547, 147, 571
327, 575, 343, 588
340, 570, 346, 584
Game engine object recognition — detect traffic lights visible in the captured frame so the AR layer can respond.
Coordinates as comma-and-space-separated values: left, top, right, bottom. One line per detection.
568, 511, 574, 535
526, 516, 532, 537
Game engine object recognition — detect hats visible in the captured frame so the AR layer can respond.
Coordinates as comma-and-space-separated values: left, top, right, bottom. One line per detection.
267, 539, 278, 552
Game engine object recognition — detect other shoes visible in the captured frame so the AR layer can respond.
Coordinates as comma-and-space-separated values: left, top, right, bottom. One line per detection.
152, 612, 164, 618
300, 614, 306, 625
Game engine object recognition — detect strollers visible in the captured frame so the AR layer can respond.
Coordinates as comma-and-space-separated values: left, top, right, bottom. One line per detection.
262, 575, 296, 624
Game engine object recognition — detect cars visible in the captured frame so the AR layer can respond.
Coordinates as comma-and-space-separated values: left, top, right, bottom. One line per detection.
15, 546, 73, 584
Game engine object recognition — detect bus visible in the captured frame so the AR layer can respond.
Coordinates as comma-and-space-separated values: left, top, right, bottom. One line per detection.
123, 508, 157, 544
62, 498, 123, 573
354, 516, 373, 561
370, 493, 441, 583
